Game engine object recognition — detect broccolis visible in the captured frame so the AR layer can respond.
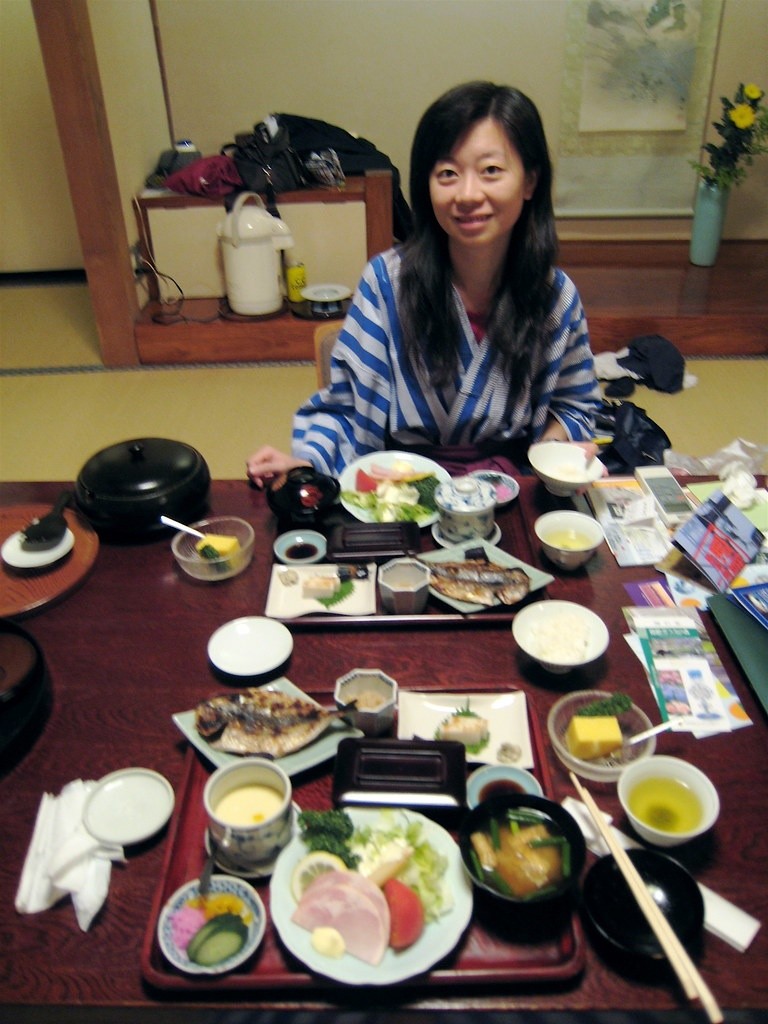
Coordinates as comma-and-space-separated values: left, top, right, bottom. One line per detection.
297, 810, 358, 868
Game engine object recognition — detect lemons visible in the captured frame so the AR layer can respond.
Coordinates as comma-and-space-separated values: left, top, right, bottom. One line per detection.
292, 851, 347, 902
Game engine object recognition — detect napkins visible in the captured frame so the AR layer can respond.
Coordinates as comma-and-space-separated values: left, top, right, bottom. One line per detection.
14, 777, 129, 933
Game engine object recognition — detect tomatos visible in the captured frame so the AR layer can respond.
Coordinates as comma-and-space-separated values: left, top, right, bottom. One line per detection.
385, 879, 423, 948
356, 468, 377, 493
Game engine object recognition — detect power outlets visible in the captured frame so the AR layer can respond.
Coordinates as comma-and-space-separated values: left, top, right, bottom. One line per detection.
127, 248, 145, 283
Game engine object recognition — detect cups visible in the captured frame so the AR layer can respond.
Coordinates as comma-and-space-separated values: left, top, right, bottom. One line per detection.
202, 757, 292, 873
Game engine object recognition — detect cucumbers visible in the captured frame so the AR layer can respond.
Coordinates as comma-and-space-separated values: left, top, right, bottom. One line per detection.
187, 914, 247, 964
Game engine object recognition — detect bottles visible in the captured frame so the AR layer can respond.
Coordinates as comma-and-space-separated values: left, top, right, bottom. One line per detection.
285, 262, 307, 303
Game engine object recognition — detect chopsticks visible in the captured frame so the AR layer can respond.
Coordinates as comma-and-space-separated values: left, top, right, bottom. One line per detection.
568, 772, 723, 1024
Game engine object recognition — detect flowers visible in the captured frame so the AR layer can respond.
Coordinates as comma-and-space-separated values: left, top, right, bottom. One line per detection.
686, 82, 768, 194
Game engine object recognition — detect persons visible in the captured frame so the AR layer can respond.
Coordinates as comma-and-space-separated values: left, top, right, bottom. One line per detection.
245, 80, 600, 488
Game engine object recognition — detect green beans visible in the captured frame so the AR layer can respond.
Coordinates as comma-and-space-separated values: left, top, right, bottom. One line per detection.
471, 808, 571, 900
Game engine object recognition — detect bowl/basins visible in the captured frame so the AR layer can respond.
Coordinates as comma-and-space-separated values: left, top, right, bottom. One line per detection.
301, 284, 352, 320
578, 848, 704, 981
434, 477, 498, 542
527, 441, 605, 496
511, 600, 610, 674
534, 509, 605, 571
170, 515, 257, 582
617, 754, 720, 849
377, 557, 432, 615
459, 792, 588, 916
547, 688, 657, 783
156, 875, 267, 976
333, 668, 398, 739
264, 465, 340, 528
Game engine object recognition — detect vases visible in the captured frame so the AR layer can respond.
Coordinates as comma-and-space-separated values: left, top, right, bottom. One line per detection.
688, 181, 731, 266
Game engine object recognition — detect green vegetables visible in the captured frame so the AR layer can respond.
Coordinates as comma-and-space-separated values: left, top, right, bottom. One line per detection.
340, 490, 433, 523
346, 821, 453, 922
579, 692, 632, 716
200, 546, 228, 572
315, 580, 354, 608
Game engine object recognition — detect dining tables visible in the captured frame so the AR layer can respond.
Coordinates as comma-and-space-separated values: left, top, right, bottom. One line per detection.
0, 476, 768, 1024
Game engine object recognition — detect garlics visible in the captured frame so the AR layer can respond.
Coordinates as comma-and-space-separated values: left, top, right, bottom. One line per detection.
309, 926, 345, 957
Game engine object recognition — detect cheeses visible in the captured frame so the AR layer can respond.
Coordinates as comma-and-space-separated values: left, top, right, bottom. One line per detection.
195, 532, 243, 569
566, 715, 623, 758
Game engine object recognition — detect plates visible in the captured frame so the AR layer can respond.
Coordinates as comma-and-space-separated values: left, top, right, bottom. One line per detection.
267, 807, 475, 986
0, 502, 100, 619
205, 800, 313, 880
414, 538, 556, 614
273, 529, 327, 564
338, 450, 454, 528
466, 763, 544, 810
462, 470, 521, 508
263, 562, 377, 620
207, 615, 294, 676
171, 675, 365, 777
396, 690, 535, 770
0, 527, 76, 570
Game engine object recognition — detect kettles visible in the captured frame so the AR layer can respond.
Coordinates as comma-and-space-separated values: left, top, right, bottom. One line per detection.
215, 192, 294, 316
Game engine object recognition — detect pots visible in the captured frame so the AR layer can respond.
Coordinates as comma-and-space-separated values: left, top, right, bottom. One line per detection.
73, 437, 212, 548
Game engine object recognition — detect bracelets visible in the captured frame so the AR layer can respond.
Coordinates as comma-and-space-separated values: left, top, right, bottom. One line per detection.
546, 439, 559, 442
